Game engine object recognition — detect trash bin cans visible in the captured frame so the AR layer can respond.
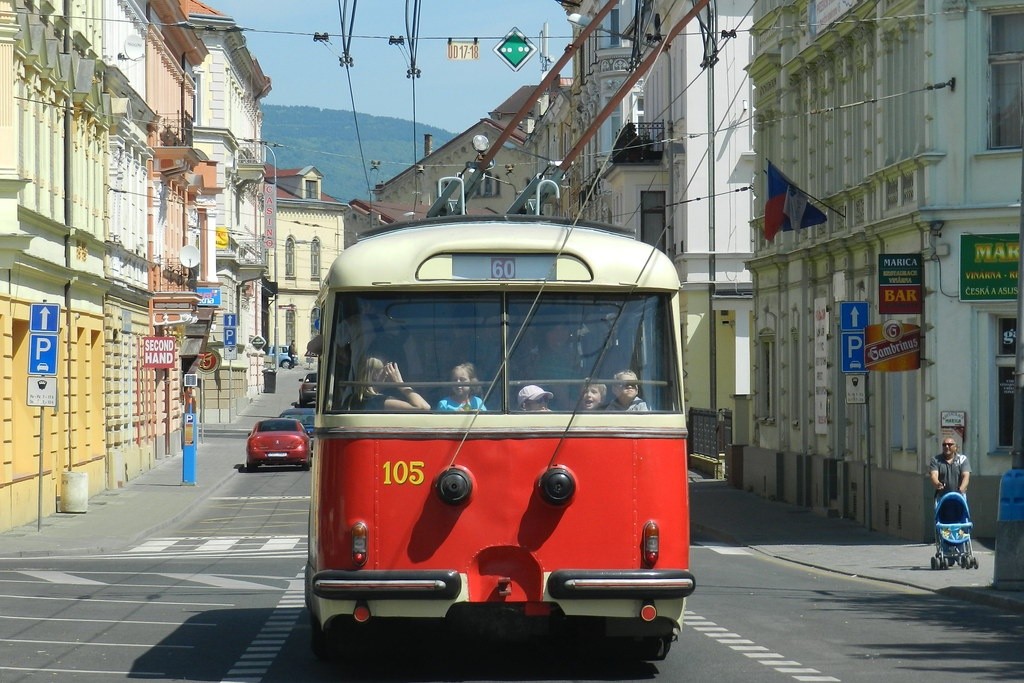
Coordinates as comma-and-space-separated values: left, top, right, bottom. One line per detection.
61, 471, 88, 514
262, 368, 278, 393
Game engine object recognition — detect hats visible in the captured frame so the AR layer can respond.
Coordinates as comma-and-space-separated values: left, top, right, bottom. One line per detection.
518, 385, 554, 405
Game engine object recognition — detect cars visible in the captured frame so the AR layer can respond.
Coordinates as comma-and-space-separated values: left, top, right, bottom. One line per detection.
246, 418, 313, 470
267, 346, 299, 369
297, 371, 319, 407
278, 408, 316, 435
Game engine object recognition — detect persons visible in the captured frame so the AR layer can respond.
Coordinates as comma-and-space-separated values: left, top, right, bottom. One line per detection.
929, 437, 972, 566
307, 297, 632, 409
288, 340, 297, 369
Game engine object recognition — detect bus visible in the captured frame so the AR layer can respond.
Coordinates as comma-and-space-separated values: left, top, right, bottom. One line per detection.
304, 214, 695, 660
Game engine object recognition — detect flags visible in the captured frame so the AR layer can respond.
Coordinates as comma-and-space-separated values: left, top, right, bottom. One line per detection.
764, 160, 829, 242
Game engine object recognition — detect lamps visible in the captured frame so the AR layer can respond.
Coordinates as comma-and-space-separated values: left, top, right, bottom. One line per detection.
930, 220, 944, 238
486, 158, 496, 171
472, 135, 489, 161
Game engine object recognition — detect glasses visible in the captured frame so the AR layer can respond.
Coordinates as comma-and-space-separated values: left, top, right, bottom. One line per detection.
942, 443, 954, 446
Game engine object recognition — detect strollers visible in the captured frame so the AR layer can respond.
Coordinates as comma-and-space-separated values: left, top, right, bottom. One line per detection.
930, 482, 980, 570
